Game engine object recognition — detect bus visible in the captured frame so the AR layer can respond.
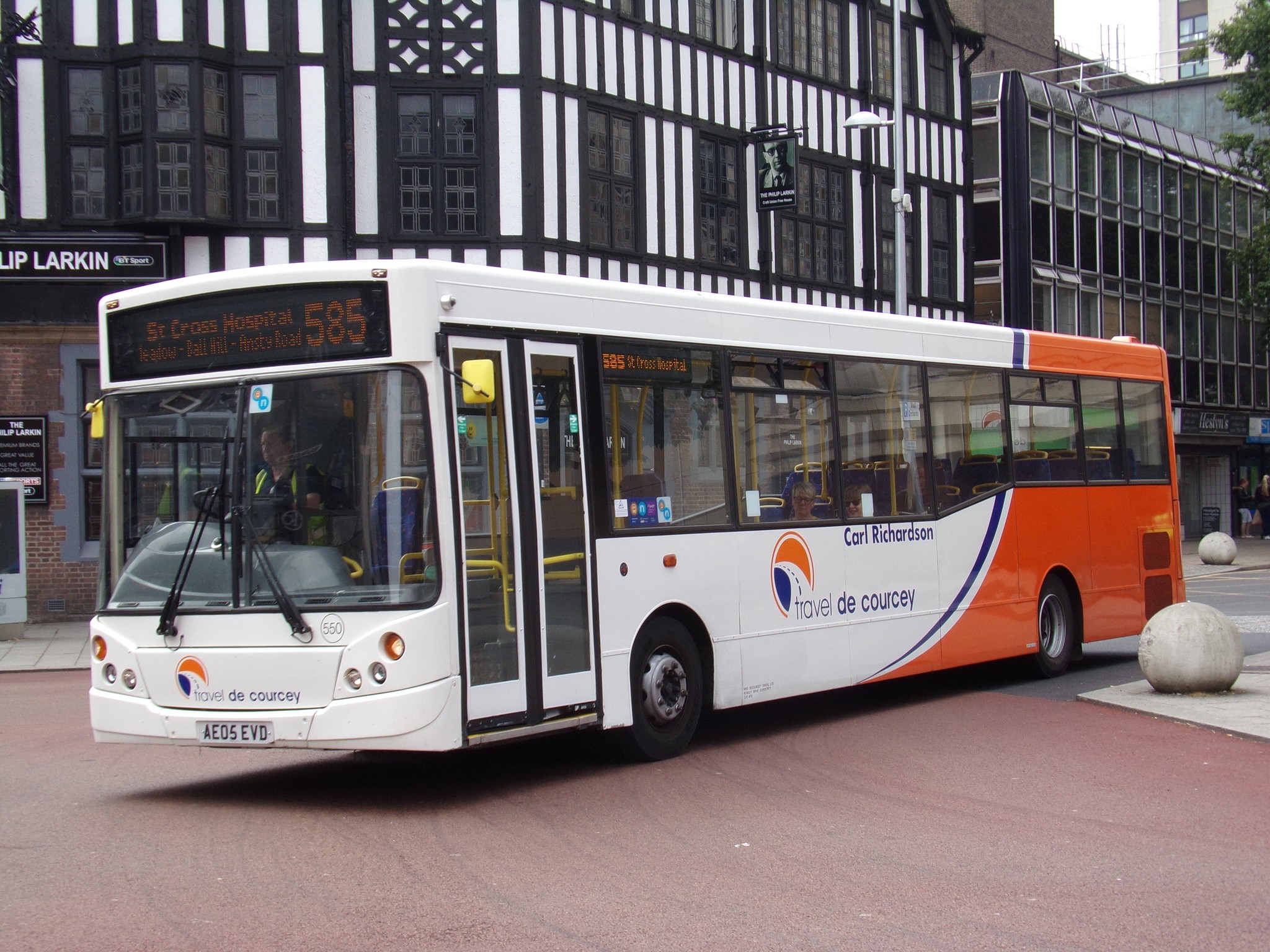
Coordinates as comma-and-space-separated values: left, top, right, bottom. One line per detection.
83, 260, 1188, 752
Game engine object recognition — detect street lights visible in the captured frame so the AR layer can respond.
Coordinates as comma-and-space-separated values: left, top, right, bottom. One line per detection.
844, 110, 908, 316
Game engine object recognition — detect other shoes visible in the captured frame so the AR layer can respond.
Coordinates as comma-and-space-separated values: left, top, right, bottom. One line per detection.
1241, 536, 1246, 538
1246, 535, 1254, 538
1263, 535, 1270, 539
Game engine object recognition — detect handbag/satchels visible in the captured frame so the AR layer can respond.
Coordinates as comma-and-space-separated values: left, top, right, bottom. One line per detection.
1251, 508, 1262, 526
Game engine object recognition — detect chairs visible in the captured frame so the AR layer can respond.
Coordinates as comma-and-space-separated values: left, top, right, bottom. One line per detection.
625, 448, 1135, 529
364, 477, 423, 583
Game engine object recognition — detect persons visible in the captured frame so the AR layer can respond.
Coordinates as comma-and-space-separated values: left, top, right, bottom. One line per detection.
896, 457, 951, 515
758, 141, 795, 188
255, 423, 329, 541
780, 481, 822, 521
844, 483, 872, 518
1232, 475, 1270, 539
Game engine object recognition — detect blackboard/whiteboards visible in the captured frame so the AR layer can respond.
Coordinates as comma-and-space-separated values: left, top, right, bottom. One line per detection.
1201, 507, 1220, 537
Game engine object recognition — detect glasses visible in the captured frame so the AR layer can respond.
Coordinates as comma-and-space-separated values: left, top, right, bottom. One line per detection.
844, 499, 862, 507
794, 496, 814, 504
